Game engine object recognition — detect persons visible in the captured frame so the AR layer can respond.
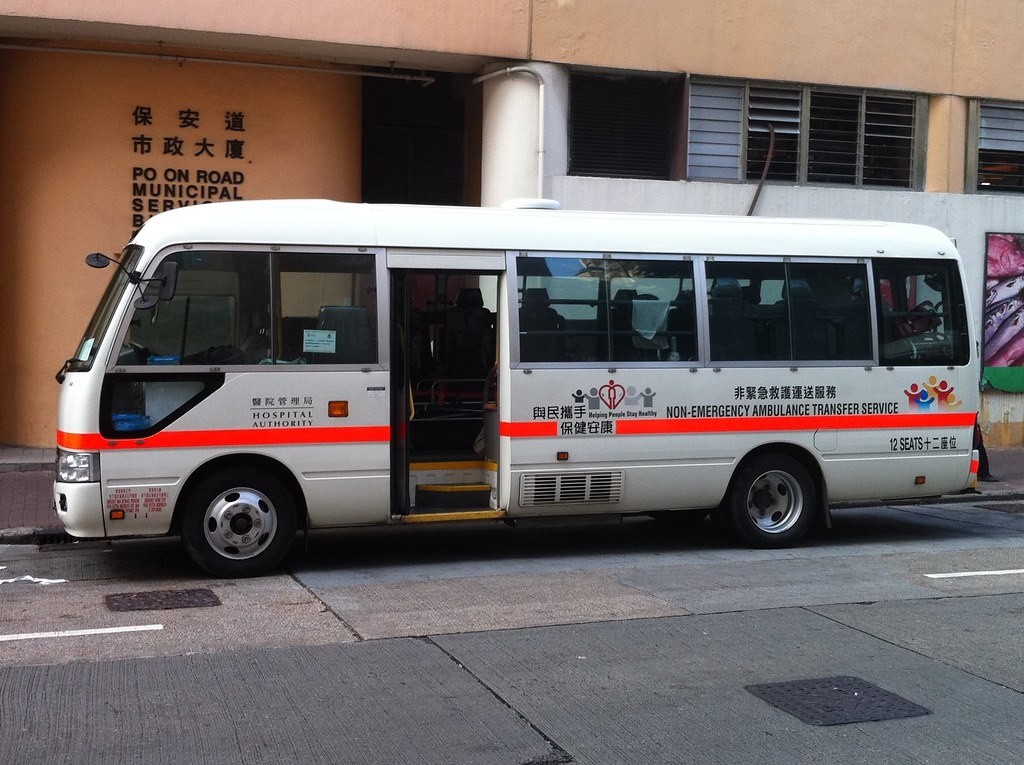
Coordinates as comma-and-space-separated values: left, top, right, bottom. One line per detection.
978, 435, 1000, 481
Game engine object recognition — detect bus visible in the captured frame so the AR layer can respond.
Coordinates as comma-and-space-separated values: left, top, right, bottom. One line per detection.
53, 196, 986, 579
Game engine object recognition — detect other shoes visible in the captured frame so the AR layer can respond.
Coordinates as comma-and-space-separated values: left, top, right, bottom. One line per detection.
977, 474, 999, 482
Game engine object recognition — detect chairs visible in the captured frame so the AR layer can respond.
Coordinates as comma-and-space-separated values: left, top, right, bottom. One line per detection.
410, 378, 496, 419
431, 288, 495, 378
611, 279, 896, 359
237, 310, 270, 362
279, 306, 376, 365
519, 287, 566, 362
